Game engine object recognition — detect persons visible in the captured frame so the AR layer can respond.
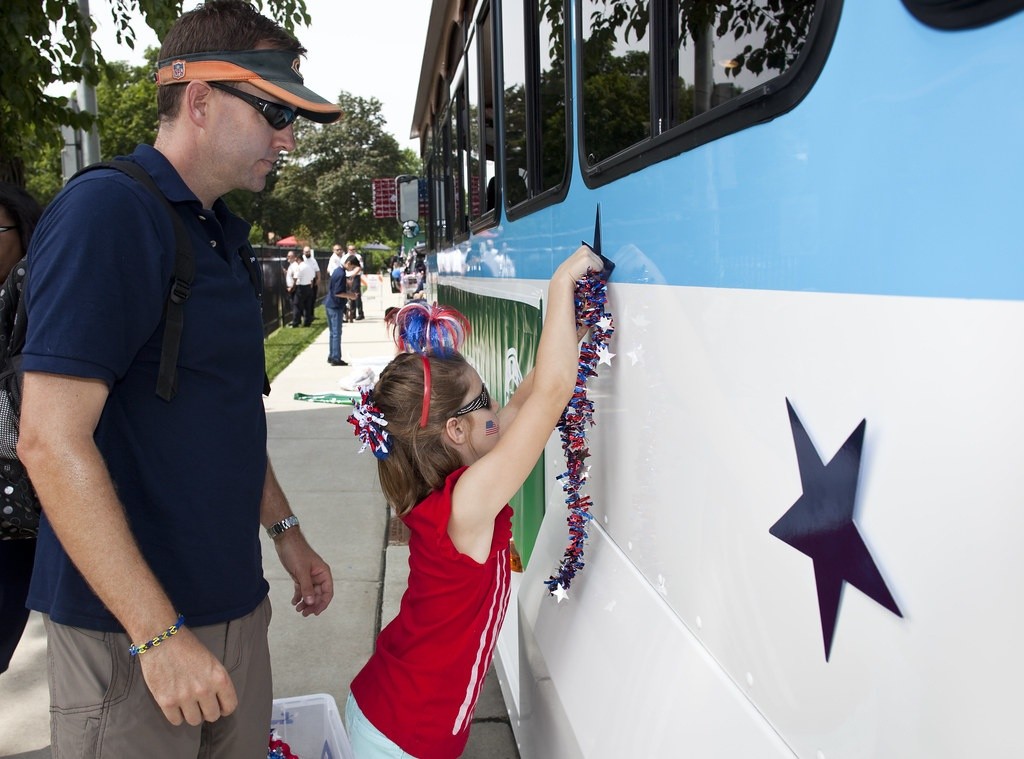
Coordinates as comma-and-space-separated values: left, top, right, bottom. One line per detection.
0, 0, 368, 759
344, 245, 603, 759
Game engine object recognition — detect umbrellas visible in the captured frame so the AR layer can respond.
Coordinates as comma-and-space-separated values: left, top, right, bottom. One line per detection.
266, 237, 308, 247
363, 240, 391, 252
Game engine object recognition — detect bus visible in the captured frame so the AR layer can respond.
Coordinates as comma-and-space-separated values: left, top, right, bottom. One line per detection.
395, 0, 1022, 759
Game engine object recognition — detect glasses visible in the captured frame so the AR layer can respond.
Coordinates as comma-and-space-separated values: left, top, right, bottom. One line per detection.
445, 384, 492, 425
207, 81, 301, 131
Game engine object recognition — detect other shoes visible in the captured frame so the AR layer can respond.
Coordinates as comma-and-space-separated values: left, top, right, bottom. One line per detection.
348, 315, 364, 320
328, 358, 348, 365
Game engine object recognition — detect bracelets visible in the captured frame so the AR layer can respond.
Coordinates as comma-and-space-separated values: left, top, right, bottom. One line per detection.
266, 515, 300, 538
129, 610, 185, 654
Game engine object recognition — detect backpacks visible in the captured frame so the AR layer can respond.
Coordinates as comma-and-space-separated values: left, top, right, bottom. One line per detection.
0, 160, 270, 542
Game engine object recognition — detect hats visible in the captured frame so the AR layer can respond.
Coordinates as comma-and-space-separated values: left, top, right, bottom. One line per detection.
155, 48, 344, 124
